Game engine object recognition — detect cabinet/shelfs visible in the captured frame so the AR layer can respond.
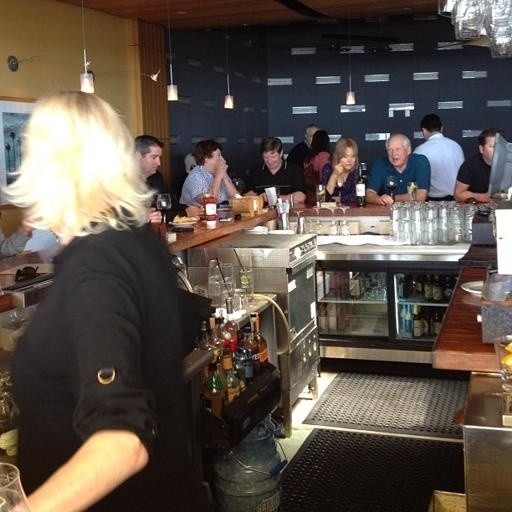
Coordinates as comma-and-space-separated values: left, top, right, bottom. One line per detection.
196, 290, 291, 458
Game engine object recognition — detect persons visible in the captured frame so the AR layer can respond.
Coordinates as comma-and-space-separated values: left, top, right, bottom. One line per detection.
145, 208, 163, 225
303, 130, 331, 207
413, 113, 465, 201
135, 134, 204, 227
364, 133, 431, 206
0, 88, 215, 512
286, 125, 320, 171
0, 210, 38, 256
177, 139, 240, 209
320, 137, 364, 204
453, 128, 506, 203
241, 137, 306, 206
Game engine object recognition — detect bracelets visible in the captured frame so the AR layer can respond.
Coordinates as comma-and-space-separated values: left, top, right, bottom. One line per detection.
392, 194, 395, 203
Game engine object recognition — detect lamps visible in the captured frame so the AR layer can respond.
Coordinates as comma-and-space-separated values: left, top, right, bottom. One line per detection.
92, 67, 163, 83
6, 50, 99, 73
162, 1, 180, 102
75, 1, 95, 94
223, 25, 235, 112
341, 0, 358, 107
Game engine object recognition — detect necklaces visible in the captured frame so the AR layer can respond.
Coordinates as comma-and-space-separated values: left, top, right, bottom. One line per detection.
340, 173, 346, 184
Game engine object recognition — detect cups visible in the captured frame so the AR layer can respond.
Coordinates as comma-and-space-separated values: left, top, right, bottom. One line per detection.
407, 180, 417, 201
191, 257, 256, 313
316, 185, 326, 203
389, 203, 474, 246
440, 0, 512, 60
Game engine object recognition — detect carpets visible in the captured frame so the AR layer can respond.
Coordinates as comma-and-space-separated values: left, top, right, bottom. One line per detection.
299, 367, 474, 443
268, 423, 469, 511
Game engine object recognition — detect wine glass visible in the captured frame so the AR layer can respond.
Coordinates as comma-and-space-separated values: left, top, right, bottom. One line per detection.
386, 176, 400, 197
370, 273, 387, 301
295, 206, 351, 237
154, 193, 173, 224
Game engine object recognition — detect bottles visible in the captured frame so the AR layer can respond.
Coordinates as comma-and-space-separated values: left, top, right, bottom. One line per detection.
355, 165, 366, 207
399, 274, 455, 339
194, 315, 269, 416
348, 271, 372, 300
202, 195, 217, 228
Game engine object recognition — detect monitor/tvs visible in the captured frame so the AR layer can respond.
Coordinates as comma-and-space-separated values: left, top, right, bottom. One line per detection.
488, 133, 512, 197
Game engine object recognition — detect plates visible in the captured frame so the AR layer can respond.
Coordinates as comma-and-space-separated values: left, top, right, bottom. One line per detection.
170, 221, 198, 230
461, 280, 512, 298
461, 293, 512, 307
